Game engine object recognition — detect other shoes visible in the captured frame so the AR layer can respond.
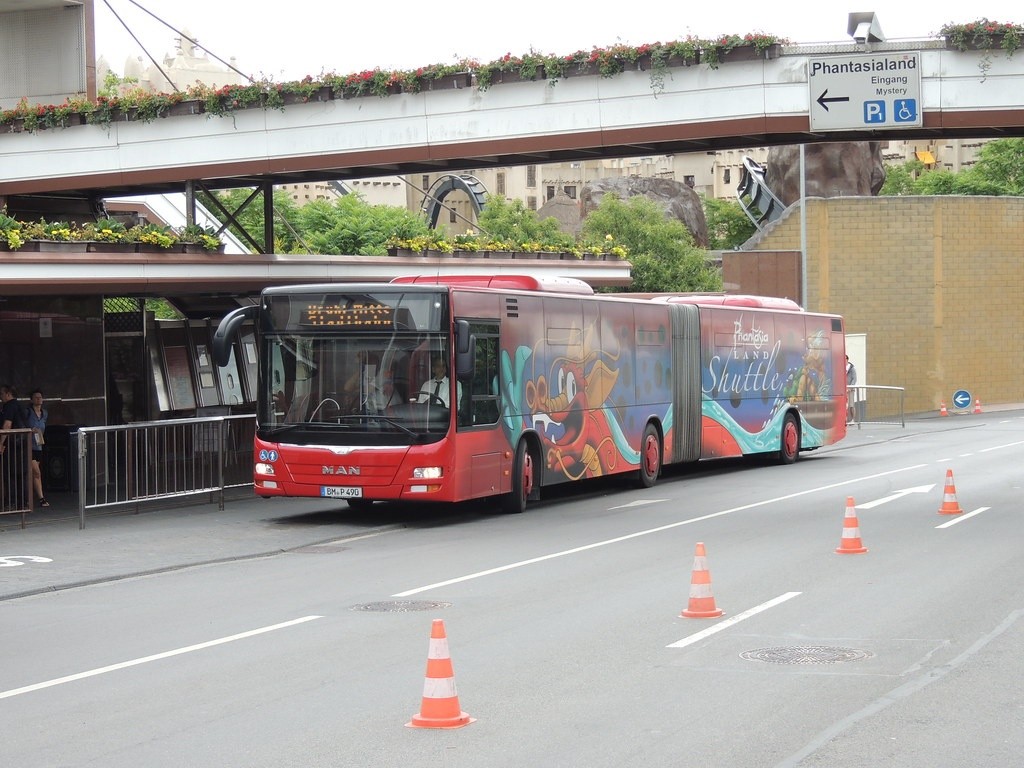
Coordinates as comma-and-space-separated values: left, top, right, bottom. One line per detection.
40, 498, 50, 507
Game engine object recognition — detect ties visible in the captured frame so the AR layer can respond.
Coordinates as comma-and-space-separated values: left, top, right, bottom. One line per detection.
433, 381, 442, 405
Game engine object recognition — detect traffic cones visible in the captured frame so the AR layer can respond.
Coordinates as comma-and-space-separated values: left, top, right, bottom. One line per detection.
940, 399, 950, 417
973, 395, 981, 414
833, 495, 869, 554
404, 619, 478, 730
679, 541, 727, 619
936, 470, 964, 515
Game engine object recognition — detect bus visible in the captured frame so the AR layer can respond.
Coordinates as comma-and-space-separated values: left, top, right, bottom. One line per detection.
208, 271, 848, 517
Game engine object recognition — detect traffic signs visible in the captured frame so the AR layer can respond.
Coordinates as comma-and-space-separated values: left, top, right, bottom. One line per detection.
806, 50, 922, 132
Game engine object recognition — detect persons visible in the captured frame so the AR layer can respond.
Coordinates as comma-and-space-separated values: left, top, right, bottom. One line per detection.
0, 383, 22, 509
418, 357, 462, 414
346, 352, 401, 408
845, 356, 855, 425
20, 391, 49, 507
